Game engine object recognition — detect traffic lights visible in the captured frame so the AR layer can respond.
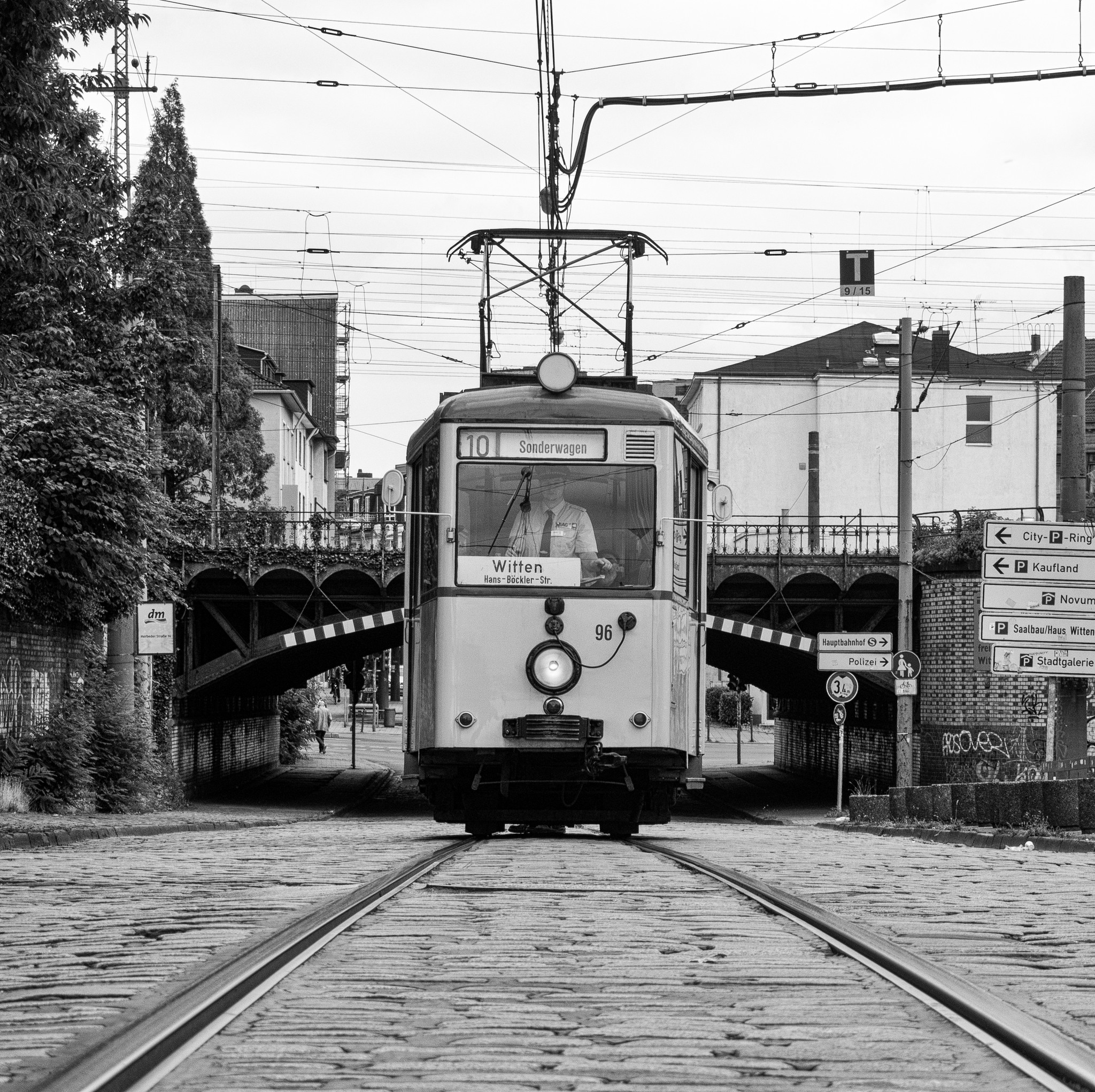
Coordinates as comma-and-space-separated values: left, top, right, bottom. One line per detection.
727, 673, 737, 690
739, 680, 747, 691
383, 649, 390, 670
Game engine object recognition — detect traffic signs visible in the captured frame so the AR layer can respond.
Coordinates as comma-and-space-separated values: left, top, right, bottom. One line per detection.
981, 551, 1095, 583
816, 632, 893, 653
982, 519, 1095, 551
817, 651, 894, 672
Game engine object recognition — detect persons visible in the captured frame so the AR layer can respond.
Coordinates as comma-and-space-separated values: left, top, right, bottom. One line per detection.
506, 463, 611, 585
327, 666, 341, 703
593, 549, 626, 588
311, 700, 332, 754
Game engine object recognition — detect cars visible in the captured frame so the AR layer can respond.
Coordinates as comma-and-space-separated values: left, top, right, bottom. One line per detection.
388, 665, 403, 695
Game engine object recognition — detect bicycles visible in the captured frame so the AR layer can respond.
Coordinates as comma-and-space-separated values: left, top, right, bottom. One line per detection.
325, 675, 342, 705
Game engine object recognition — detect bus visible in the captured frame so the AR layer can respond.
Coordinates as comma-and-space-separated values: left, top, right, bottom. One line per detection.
381, 229, 734, 842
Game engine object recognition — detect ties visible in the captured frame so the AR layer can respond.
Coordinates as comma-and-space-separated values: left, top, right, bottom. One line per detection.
539, 510, 553, 558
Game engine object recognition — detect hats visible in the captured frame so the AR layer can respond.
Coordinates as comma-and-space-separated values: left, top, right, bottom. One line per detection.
534, 465, 569, 482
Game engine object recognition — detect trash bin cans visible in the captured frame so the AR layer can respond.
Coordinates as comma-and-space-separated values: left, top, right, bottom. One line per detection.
384, 708, 396, 727
